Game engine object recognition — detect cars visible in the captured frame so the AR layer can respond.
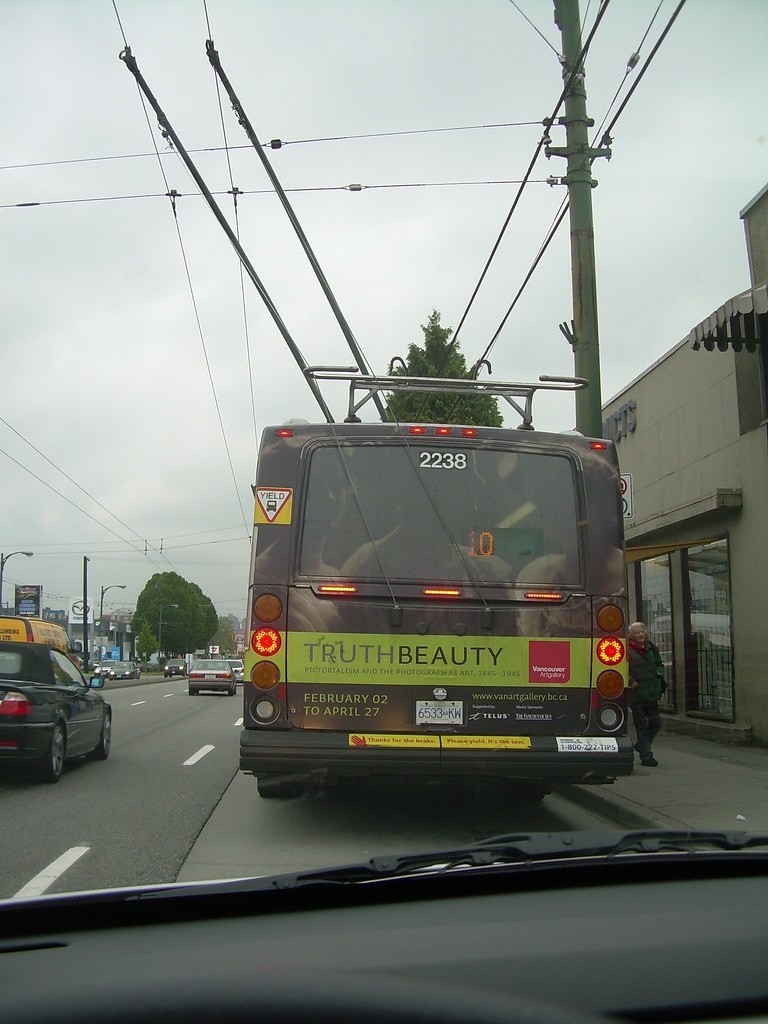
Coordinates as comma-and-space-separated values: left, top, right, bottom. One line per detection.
224, 659, 245, 684
164, 658, 189, 678
0, 641, 112, 781
93, 659, 118, 679
187, 659, 237, 697
108, 661, 141, 679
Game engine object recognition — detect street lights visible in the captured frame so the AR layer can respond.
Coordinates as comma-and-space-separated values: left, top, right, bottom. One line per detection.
98, 585, 127, 676
158, 605, 178, 672
0, 550, 34, 615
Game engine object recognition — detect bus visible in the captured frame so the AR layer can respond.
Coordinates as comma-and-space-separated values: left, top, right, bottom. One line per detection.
239, 357, 635, 802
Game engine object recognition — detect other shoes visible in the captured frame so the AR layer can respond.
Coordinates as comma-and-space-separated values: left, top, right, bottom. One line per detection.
641, 758, 658, 766
635, 743, 653, 754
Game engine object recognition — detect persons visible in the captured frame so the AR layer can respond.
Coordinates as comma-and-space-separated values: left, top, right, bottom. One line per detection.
251, 436, 365, 634
628, 622, 669, 767
513, 459, 628, 637
331, 447, 520, 638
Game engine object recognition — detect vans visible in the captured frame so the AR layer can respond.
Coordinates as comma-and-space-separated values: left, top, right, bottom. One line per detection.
0, 612, 83, 686
